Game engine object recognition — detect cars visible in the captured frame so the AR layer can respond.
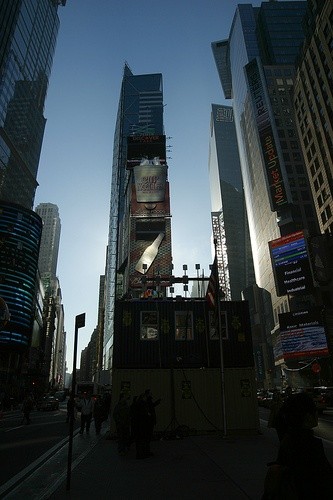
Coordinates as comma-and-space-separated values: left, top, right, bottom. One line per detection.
256, 386, 333, 418
36, 390, 67, 411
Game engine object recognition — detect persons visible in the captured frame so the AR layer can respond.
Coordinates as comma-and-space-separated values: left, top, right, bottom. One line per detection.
271, 393, 333, 500
67, 388, 161, 453
21, 392, 33, 424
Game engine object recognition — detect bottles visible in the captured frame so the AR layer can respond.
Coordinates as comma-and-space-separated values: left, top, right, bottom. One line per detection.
135, 233, 164, 274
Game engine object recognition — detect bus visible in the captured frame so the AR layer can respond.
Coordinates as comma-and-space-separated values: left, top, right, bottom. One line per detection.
74, 381, 104, 405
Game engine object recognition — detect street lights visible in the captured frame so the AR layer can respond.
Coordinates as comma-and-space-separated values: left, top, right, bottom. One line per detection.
67, 313, 86, 463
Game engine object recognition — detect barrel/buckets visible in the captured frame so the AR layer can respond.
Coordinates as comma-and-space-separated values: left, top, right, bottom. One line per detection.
133, 166, 167, 202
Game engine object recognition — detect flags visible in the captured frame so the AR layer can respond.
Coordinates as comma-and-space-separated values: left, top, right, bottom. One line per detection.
205, 258, 219, 309
